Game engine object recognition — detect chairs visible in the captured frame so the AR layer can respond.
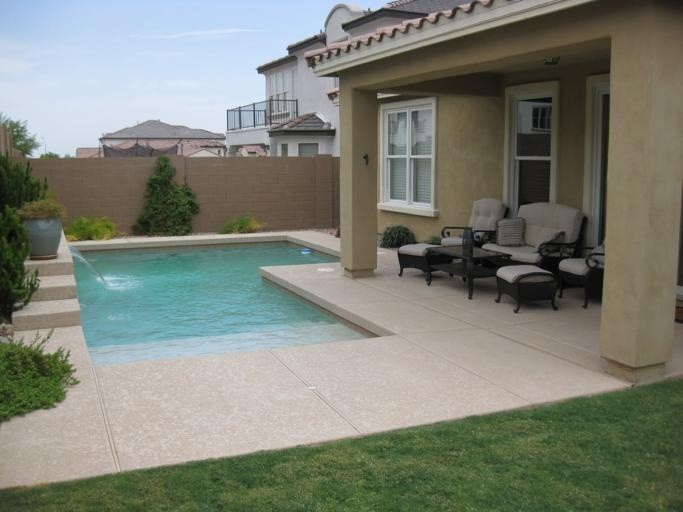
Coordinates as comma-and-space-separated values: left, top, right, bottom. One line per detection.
397, 199, 607, 314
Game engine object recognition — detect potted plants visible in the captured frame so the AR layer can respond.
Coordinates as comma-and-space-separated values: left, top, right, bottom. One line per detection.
21, 199, 62, 260
